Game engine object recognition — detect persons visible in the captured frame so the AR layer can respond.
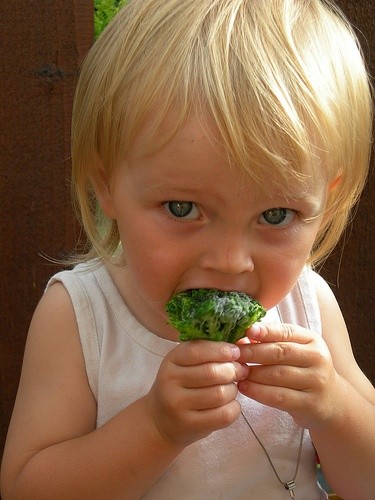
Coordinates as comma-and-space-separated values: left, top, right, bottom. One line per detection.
0, 0, 375, 500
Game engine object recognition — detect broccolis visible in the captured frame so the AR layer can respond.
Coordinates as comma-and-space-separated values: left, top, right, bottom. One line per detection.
163, 287, 267, 345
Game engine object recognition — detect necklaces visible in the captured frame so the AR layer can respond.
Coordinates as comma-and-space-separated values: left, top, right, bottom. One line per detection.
240, 411, 304, 500
